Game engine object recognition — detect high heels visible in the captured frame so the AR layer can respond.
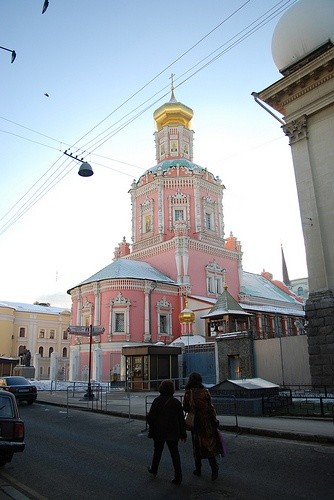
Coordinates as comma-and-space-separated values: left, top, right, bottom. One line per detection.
148, 465, 157, 476
172, 477, 182, 485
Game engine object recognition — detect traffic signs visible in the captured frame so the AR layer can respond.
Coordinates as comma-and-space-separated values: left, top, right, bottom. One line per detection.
92, 326, 106, 335
65, 326, 89, 334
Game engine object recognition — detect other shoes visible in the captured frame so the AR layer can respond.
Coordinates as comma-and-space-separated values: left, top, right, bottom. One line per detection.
212, 466, 218, 479
193, 470, 201, 476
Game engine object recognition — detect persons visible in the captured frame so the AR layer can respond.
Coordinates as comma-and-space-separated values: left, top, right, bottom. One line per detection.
145, 380, 188, 486
183, 372, 222, 481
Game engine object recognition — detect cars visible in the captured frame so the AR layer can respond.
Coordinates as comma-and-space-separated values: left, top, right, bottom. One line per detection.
0, 390, 25, 469
0, 376, 38, 405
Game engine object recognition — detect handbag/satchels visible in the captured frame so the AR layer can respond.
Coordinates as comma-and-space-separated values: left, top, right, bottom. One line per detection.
217, 429, 227, 459
185, 389, 194, 428
